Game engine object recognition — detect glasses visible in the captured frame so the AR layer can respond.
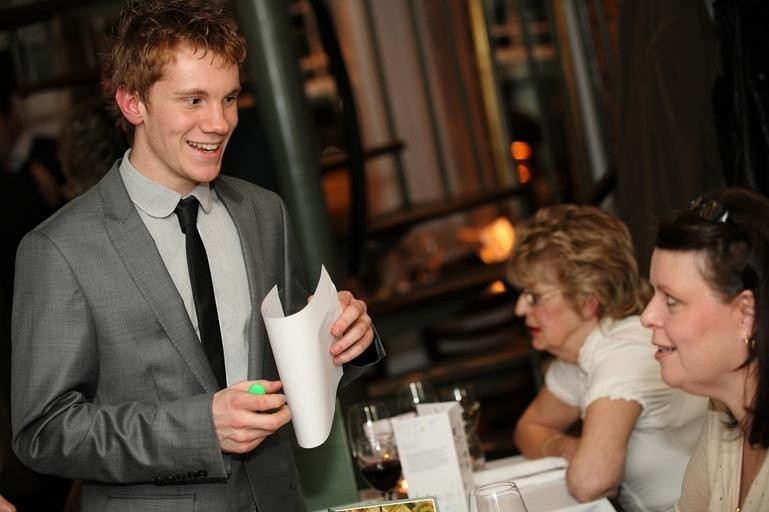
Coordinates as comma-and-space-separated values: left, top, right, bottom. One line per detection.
685, 192, 753, 251
523, 287, 570, 306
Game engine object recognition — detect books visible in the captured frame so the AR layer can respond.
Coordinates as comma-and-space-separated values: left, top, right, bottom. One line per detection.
328, 496, 440, 512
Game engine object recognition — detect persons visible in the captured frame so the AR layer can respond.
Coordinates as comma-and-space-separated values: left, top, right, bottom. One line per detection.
10, 2, 388, 512
640, 188, 768, 512
504, 187, 711, 511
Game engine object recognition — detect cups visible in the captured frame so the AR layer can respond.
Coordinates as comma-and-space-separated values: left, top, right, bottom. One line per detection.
470, 482, 529, 512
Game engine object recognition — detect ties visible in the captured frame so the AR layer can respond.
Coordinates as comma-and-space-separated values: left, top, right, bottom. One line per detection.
173, 195, 227, 392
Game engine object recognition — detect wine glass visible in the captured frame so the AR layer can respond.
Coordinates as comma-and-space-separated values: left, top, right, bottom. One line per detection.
347, 403, 401, 501
